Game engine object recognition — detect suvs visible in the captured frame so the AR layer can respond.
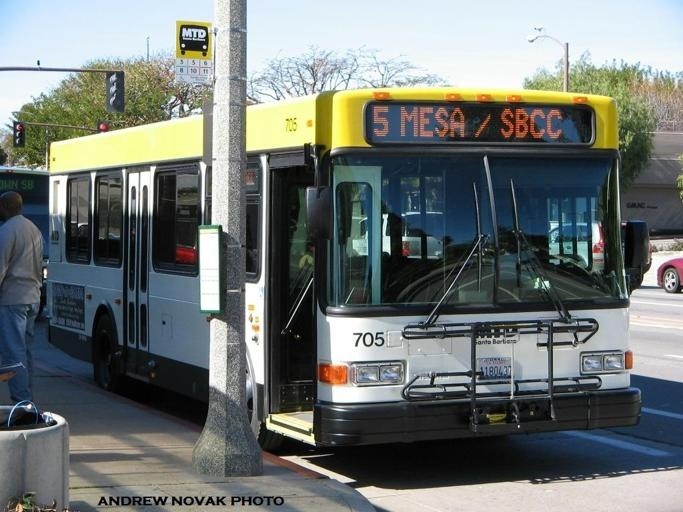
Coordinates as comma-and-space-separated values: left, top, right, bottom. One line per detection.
549, 222, 651, 287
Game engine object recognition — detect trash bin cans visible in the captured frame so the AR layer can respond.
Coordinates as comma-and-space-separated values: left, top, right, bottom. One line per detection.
0, 405, 69, 512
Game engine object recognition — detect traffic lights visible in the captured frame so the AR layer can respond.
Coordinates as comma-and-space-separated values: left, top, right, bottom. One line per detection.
49, 86, 642, 454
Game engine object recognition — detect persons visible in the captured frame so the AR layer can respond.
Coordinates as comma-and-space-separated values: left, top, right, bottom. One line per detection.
0, 190, 43, 405
298, 237, 315, 272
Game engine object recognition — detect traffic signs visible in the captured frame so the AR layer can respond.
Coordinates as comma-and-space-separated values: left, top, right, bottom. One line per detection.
14, 124, 22, 145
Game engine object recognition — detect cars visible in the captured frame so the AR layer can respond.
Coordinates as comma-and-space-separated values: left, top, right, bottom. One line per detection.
656, 256, 683, 293
351, 211, 441, 262
70, 221, 88, 241
289, 222, 319, 241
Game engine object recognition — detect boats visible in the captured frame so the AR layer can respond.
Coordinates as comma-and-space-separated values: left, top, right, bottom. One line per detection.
99, 123, 106, 134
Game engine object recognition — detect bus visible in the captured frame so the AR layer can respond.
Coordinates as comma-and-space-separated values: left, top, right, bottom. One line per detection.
0, 167, 49, 314
175, 204, 197, 264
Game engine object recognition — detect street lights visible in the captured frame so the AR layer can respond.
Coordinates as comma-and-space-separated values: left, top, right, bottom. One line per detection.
145, 36, 150, 63
528, 26, 570, 90
